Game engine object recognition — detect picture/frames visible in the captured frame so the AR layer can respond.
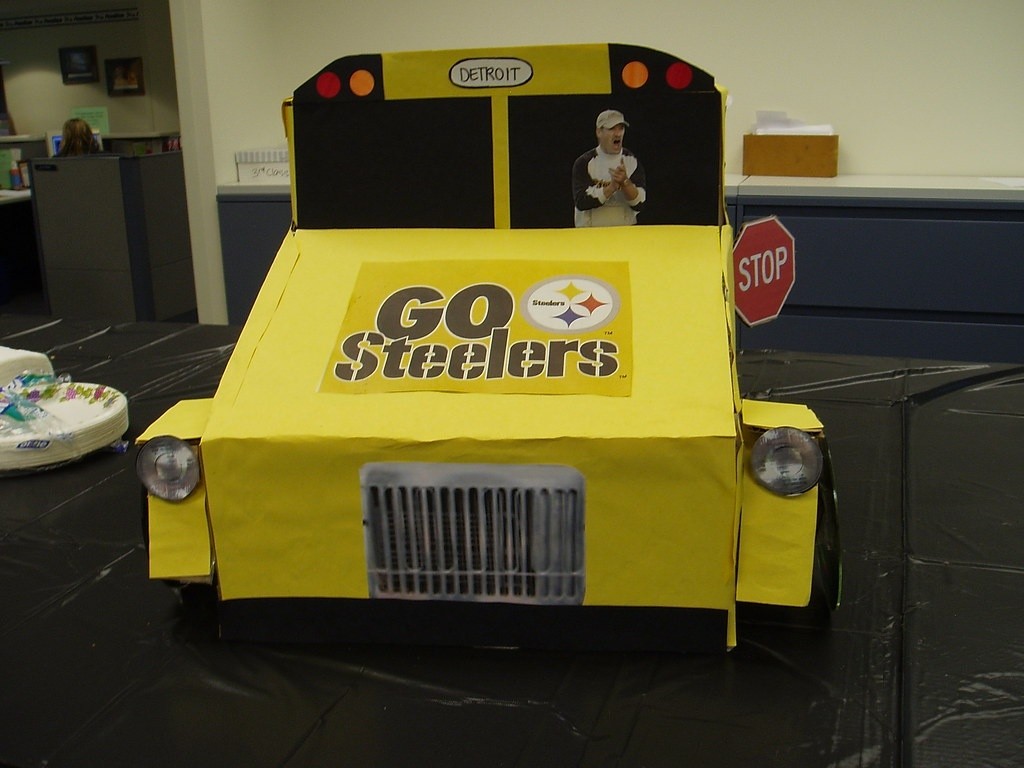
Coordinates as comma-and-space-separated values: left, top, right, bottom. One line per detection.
104, 57, 145, 97
57, 45, 101, 86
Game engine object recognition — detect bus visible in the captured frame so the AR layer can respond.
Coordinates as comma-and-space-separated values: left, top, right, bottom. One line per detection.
133, 44, 846, 654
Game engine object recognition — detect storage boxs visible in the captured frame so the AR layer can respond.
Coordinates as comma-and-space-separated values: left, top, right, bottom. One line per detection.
741, 133, 839, 177
232, 151, 291, 186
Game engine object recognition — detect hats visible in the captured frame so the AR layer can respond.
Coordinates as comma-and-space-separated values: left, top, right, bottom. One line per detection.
595, 109, 630, 129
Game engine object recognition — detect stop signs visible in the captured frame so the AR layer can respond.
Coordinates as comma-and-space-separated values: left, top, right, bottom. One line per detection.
734, 216, 796, 328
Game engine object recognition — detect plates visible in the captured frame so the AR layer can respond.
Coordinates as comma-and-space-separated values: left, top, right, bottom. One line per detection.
0, 382, 128, 469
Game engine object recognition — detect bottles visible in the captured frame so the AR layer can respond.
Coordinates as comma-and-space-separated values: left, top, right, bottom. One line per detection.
10, 161, 22, 190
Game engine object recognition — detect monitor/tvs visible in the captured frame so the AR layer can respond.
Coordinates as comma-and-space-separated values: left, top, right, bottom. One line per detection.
46, 128, 104, 158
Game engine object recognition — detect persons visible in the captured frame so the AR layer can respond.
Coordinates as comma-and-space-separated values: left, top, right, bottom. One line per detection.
52, 118, 101, 157
572, 112, 648, 229
